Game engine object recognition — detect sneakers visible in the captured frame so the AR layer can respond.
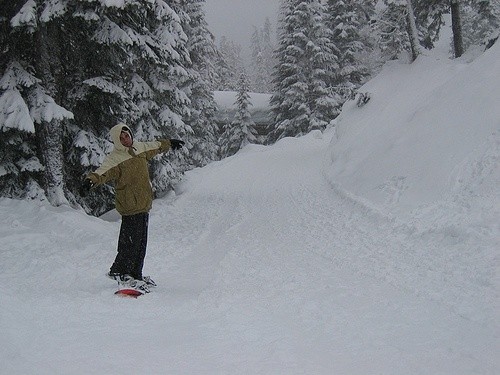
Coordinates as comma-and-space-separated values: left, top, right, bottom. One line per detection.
105, 271, 134, 286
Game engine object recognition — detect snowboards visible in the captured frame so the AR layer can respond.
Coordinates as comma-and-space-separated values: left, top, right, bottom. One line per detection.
114, 275, 152, 296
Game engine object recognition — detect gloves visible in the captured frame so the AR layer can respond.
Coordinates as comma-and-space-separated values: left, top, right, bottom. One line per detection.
79, 178, 94, 198
170, 139, 185, 151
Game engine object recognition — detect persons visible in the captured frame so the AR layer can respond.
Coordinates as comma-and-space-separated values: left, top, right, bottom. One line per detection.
84, 122, 185, 290
